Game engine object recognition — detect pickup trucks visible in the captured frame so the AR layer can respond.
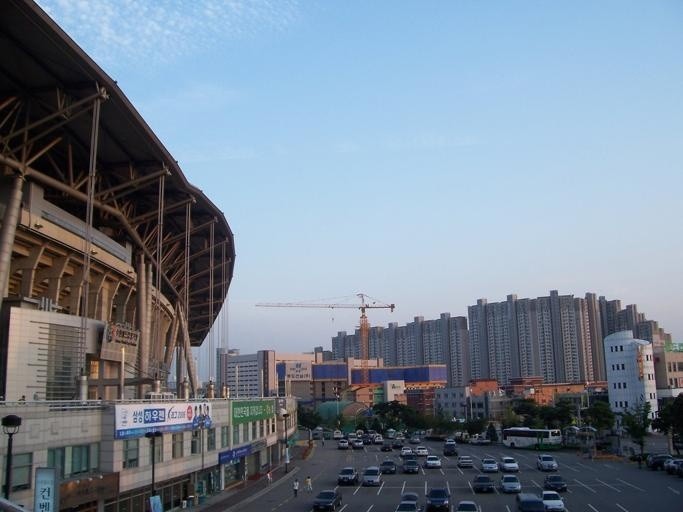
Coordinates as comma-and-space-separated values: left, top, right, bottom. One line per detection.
456, 455, 473, 468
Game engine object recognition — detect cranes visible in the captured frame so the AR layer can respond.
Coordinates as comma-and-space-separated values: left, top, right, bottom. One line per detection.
255, 289, 397, 384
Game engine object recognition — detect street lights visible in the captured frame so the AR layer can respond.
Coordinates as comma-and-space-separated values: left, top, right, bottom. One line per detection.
191, 404, 211, 488
143, 429, 162, 512
1, 414, 22, 512
282, 414, 291, 475
330, 384, 343, 427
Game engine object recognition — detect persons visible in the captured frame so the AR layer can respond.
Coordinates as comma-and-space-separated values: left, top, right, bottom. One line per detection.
197, 404, 204, 429
16, 396, 26, 405
192, 405, 199, 428
263, 470, 272, 487
637, 454, 641, 469
304, 475, 312, 495
202, 405, 211, 428
292, 478, 299, 497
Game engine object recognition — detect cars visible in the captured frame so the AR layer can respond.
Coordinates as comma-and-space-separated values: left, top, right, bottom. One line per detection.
380, 459, 397, 474
471, 475, 495, 494
312, 426, 491, 457
455, 499, 482, 512
543, 474, 567, 491
540, 491, 564, 512
424, 455, 442, 467
312, 490, 342, 512
402, 459, 420, 473
500, 475, 521, 494
397, 492, 418, 512
561, 426, 598, 433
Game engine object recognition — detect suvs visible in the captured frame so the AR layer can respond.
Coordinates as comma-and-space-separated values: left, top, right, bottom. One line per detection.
338, 466, 358, 485
644, 452, 682, 474
424, 486, 451, 511
536, 455, 558, 471
480, 459, 499, 473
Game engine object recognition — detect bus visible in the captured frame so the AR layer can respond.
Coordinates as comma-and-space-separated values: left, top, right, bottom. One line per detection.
502, 426, 563, 449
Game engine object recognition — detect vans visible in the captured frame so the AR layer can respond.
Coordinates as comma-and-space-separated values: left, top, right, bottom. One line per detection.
362, 466, 382, 486
514, 492, 543, 512
500, 456, 520, 472
